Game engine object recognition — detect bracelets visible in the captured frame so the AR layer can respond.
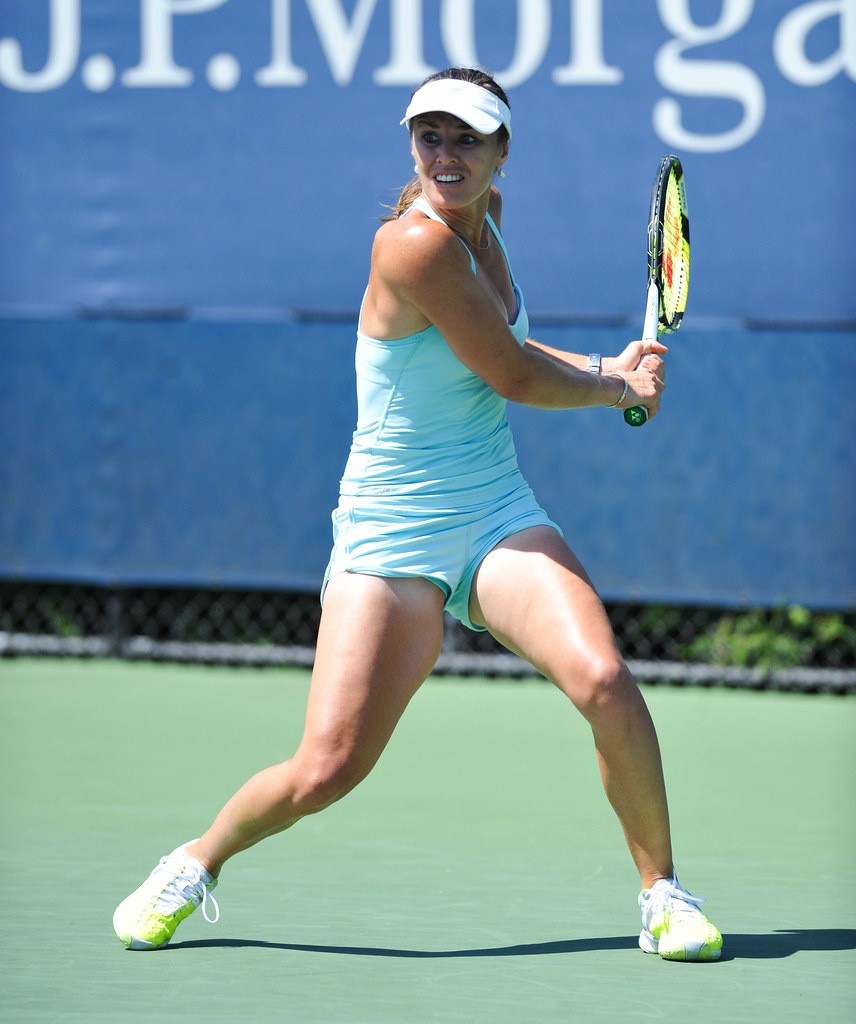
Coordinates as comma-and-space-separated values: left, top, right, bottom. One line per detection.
603, 373, 631, 410
587, 353, 602, 375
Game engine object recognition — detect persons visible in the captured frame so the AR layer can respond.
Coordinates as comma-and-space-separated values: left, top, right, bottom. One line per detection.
111, 67, 727, 965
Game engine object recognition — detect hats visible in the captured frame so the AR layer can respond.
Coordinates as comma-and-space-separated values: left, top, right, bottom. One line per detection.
400, 79, 512, 143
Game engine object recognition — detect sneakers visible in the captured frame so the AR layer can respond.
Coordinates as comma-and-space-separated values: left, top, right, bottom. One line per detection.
638, 867, 723, 962
113, 839, 219, 950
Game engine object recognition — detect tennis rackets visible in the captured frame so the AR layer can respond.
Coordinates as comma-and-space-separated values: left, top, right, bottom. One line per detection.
623, 153, 692, 428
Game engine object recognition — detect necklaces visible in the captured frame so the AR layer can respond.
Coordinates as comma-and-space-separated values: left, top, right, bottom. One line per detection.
431, 208, 491, 250
